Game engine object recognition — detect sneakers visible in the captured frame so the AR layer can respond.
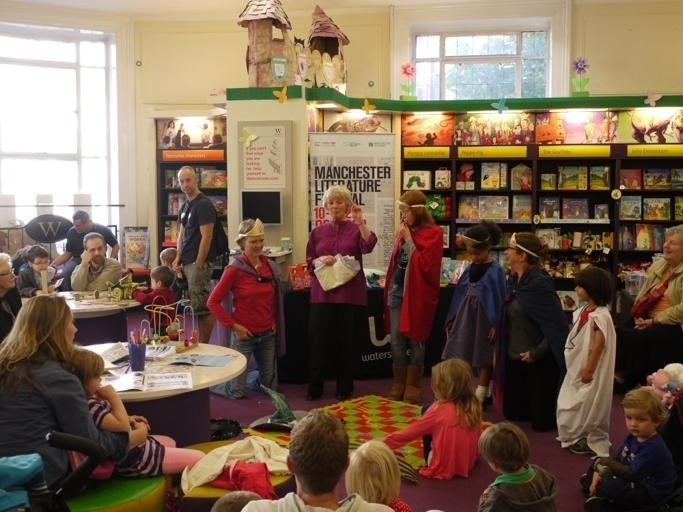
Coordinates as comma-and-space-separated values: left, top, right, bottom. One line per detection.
477, 395, 495, 411
569, 437, 596, 455
583, 496, 611, 512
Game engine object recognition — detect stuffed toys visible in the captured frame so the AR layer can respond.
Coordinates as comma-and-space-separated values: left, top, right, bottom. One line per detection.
644, 362, 683, 410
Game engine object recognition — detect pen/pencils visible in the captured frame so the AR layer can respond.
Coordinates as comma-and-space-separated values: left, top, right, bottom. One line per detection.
125, 365, 131, 374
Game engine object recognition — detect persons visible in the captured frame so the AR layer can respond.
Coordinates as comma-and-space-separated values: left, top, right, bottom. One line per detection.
628, 224, 683, 368
159, 247, 189, 314
378, 188, 445, 403
490, 231, 567, 432
0, 294, 151, 496
576, 387, 677, 510
379, 357, 482, 480
0, 251, 22, 344
48, 210, 120, 291
168, 165, 229, 313
209, 490, 263, 511
238, 408, 398, 512
69, 231, 121, 291
129, 265, 176, 336
14, 244, 57, 299
475, 419, 559, 511
553, 264, 619, 460
204, 218, 282, 400
342, 439, 413, 512
304, 184, 378, 400
63, 348, 205, 478
440, 219, 509, 413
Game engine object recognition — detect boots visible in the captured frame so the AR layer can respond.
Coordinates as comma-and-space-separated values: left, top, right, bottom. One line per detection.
386, 365, 407, 401
401, 365, 426, 403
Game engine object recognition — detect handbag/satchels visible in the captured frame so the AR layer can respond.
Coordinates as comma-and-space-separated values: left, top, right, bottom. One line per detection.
205, 221, 229, 260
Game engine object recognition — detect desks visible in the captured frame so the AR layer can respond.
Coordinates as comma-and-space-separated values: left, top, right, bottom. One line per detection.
20, 291, 142, 347
77, 340, 249, 448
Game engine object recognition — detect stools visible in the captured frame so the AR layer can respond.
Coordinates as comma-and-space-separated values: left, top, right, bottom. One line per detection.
64, 474, 167, 512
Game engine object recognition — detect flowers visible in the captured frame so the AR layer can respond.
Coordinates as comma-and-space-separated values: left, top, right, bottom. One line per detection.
569, 56, 592, 92
398, 62, 417, 96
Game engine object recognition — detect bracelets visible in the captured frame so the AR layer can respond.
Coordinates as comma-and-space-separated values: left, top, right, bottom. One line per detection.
649, 317, 654, 325
354, 220, 364, 226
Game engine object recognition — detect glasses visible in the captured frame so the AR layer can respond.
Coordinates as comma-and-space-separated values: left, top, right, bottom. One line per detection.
0, 268, 14, 276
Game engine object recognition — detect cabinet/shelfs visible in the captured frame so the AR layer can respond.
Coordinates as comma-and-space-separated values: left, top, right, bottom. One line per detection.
152, 84, 683, 326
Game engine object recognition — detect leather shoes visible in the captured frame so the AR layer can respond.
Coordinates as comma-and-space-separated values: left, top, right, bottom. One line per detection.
304, 393, 318, 402
335, 392, 349, 399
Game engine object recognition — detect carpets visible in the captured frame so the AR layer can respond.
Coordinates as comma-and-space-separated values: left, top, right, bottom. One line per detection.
239, 391, 495, 478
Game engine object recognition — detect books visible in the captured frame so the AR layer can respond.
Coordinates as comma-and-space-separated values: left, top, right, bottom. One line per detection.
169, 351, 240, 368
97, 371, 194, 392
143, 343, 176, 361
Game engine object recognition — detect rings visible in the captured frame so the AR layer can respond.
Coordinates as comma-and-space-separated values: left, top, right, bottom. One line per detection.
324, 259, 329, 262
